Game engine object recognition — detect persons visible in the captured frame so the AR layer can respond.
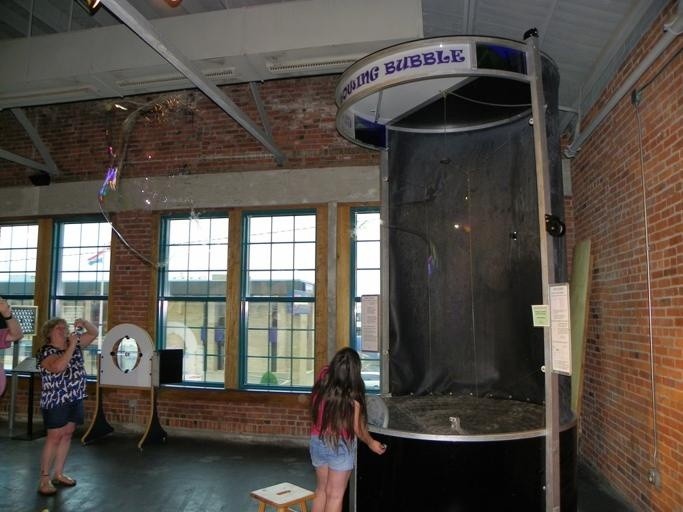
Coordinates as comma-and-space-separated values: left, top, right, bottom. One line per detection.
305, 347, 386, 512
0, 297, 25, 397
33, 317, 100, 497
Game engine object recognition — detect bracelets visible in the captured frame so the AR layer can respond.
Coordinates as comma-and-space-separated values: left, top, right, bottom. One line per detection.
4, 313, 13, 321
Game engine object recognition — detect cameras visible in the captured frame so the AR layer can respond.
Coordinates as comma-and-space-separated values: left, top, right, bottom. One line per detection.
76, 322, 85, 336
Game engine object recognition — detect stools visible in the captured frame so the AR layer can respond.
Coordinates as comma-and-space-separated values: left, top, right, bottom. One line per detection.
250, 482, 317, 512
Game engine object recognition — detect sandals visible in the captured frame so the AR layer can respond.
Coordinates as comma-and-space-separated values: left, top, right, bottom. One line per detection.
55, 474, 76, 486
40, 480, 57, 494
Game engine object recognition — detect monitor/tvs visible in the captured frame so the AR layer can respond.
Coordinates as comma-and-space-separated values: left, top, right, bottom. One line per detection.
158, 348, 184, 384
